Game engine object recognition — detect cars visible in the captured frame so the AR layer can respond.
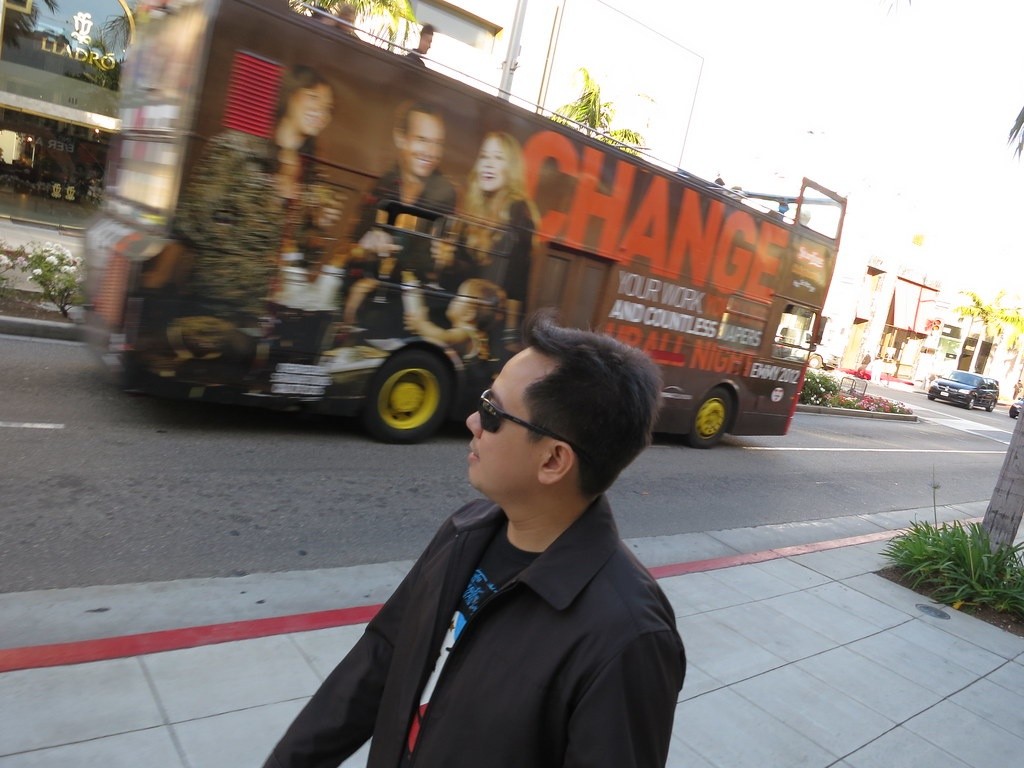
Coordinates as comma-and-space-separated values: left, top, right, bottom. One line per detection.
1008, 397, 1024, 418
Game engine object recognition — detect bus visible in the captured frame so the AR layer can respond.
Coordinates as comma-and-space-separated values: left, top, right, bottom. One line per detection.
83, 0, 847, 448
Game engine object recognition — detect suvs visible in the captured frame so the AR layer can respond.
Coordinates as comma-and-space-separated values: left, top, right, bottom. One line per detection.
927, 370, 1000, 413
776, 324, 843, 371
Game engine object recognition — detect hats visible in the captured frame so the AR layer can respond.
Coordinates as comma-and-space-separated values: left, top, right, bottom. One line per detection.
359, 230, 404, 253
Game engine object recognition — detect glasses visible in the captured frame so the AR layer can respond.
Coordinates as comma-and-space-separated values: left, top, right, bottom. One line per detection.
478, 388, 577, 453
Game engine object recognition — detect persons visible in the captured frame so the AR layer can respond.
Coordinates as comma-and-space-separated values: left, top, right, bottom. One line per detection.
409, 23, 434, 68
311, 5, 360, 38
236, 60, 542, 397
1013, 380, 1023, 401
860, 352, 870, 372
259, 306, 686, 768
